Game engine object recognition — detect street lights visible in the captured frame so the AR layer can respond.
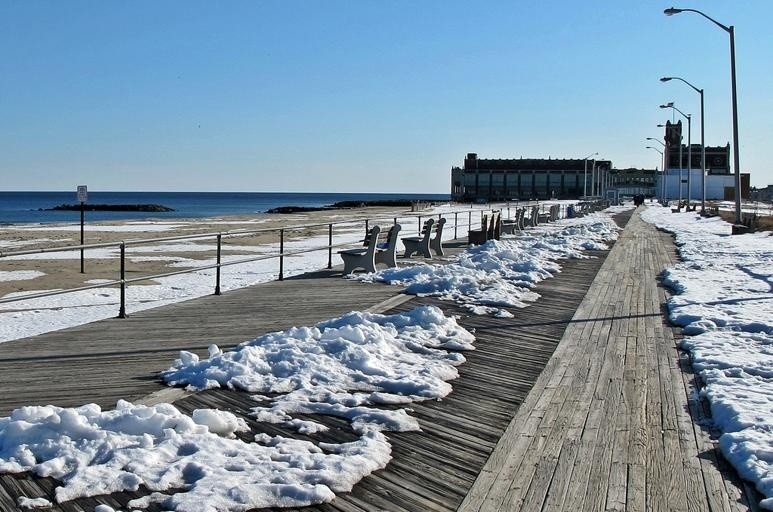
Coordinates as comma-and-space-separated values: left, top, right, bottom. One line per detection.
645, 136, 668, 205
660, 77, 707, 214
656, 123, 681, 209
583, 153, 622, 199
663, 7, 742, 226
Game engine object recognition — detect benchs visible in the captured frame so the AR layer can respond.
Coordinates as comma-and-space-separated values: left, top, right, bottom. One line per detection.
340, 224, 401, 274
401, 218, 446, 257
468, 212, 501, 246
500, 198, 610, 235
